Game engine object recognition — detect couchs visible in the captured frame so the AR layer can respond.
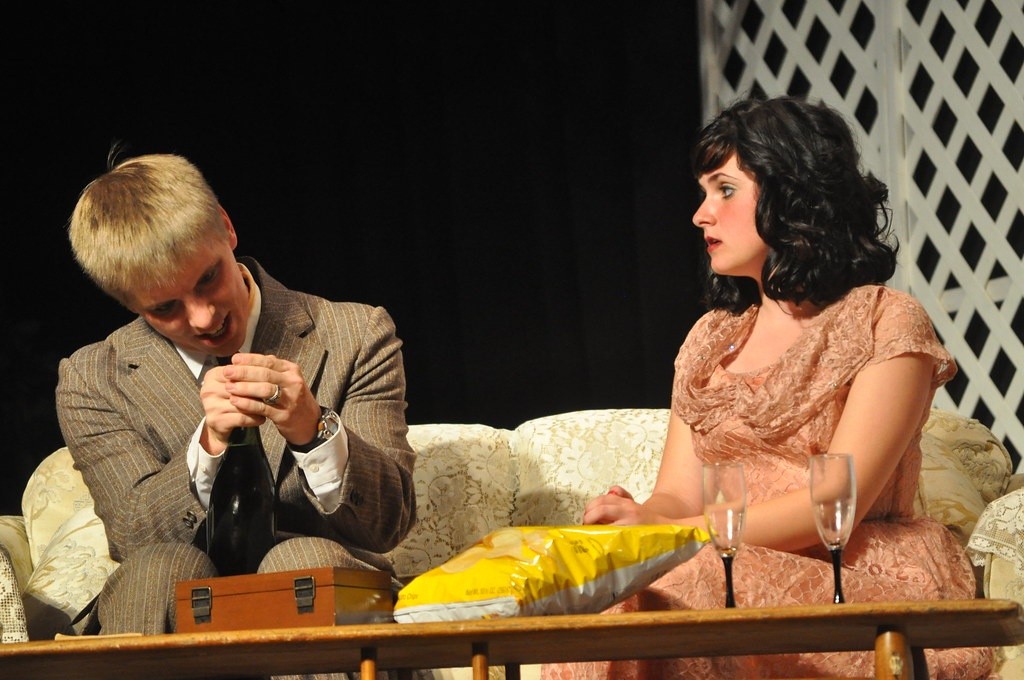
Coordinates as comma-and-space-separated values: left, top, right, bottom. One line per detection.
0, 406, 1024, 680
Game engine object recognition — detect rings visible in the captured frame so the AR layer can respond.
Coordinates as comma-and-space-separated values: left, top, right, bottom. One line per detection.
266, 384, 281, 404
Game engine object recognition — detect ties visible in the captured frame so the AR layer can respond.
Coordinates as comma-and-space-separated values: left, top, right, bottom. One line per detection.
216, 355, 232, 365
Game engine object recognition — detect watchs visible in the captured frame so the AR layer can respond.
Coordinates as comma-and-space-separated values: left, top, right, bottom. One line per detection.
286, 405, 339, 454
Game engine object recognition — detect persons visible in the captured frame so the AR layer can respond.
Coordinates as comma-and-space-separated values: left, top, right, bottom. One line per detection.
540, 96, 977, 680
55, 154, 417, 680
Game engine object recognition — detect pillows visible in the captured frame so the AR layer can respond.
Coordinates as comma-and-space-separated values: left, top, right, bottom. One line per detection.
913, 431, 984, 537
21, 508, 119, 641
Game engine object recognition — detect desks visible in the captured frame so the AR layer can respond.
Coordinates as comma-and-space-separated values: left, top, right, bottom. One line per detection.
0, 597, 1024, 680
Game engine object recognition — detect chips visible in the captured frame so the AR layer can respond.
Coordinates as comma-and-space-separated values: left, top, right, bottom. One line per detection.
442, 529, 546, 568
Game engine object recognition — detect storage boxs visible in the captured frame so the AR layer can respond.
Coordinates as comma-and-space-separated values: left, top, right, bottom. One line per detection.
174, 565, 394, 635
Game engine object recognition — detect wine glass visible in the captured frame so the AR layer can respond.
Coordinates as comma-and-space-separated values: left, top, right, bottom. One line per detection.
703, 462, 747, 608
809, 453, 857, 604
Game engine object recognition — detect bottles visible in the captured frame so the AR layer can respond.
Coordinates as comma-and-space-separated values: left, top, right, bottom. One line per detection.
206, 425, 278, 576
